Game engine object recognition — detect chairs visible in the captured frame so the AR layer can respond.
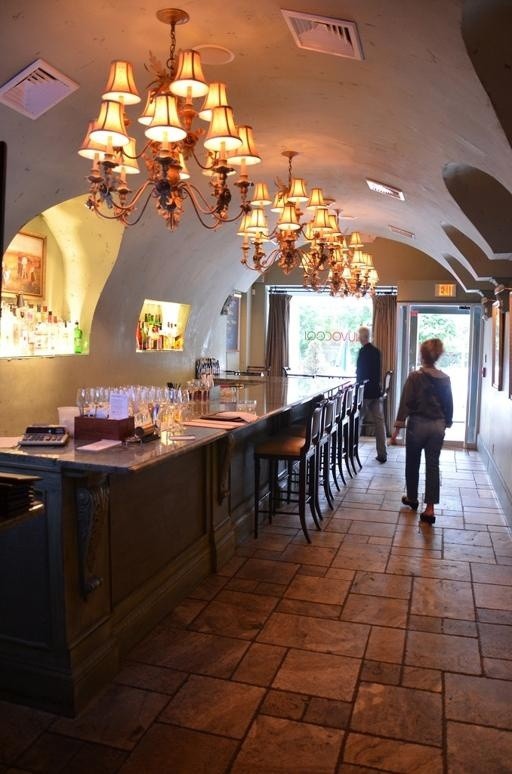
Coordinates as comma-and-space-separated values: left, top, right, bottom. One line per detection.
253, 378, 372, 545
361, 370, 394, 436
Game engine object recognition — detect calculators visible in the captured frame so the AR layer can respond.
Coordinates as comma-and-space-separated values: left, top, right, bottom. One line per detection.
18, 426, 70, 448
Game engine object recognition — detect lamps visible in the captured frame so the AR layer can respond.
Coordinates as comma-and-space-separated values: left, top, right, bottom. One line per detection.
75, 7, 263, 232
234, 149, 342, 272
301, 185, 379, 299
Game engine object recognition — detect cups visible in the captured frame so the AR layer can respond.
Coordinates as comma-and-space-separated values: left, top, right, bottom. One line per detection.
236, 399, 257, 413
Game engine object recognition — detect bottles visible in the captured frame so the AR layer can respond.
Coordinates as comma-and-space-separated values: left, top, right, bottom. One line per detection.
195, 356, 220, 377
0, 294, 84, 355
220, 381, 247, 404
137, 312, 181, 350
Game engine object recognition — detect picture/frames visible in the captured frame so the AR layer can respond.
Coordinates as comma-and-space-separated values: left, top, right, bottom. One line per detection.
508, 292, 512, 400
491, 300, 503, 391
0, 230, 47, 302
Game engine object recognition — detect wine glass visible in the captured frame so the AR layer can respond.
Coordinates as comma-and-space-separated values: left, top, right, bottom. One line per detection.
75, 384, 189, 433
185, 377, 213, 405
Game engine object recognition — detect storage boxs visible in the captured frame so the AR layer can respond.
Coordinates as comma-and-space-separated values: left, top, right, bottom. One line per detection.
73, 414, 134, 443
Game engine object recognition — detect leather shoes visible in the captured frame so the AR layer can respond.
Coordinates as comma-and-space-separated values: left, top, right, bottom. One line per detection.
402, 496, 419, 511
420, 512, 435, 523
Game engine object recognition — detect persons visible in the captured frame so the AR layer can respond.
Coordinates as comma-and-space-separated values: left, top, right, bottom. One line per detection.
389, 338, 453, 524
355, 326, 388, 464
